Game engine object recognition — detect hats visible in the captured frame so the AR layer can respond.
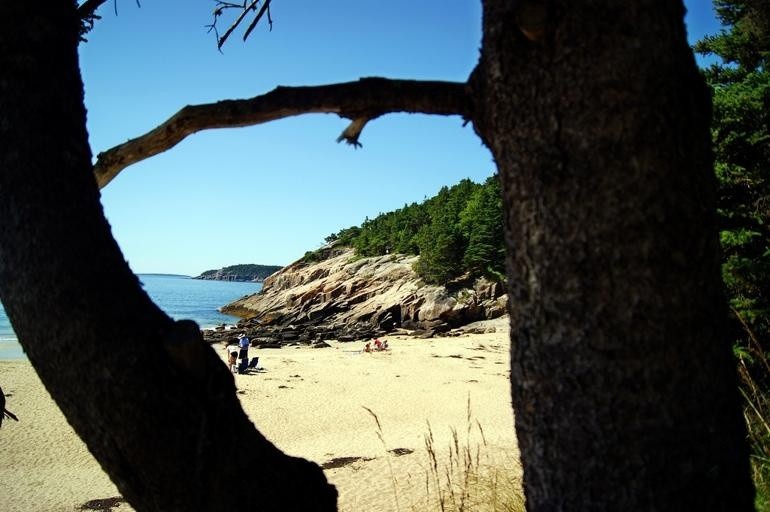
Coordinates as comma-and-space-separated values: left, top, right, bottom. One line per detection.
237, 333, 245, 340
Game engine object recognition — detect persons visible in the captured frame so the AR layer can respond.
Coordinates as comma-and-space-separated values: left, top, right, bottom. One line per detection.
238, 334, 249, 359
228, 352, 238, 373
364, 338, 388, 352
